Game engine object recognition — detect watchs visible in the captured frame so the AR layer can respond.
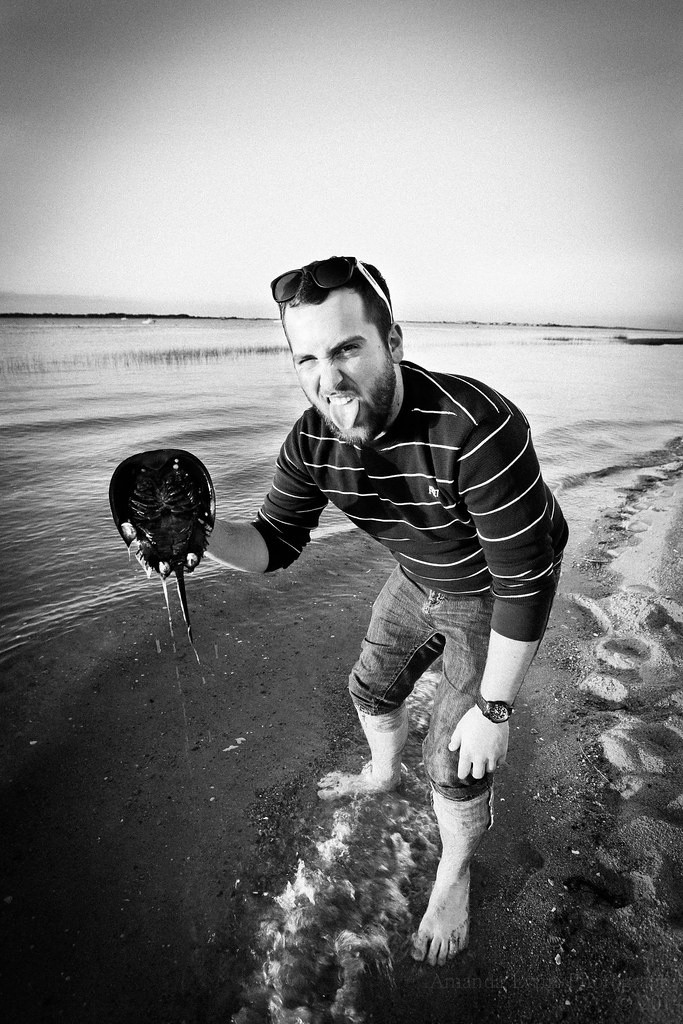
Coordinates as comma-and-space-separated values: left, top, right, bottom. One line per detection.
473, 693, 516, 723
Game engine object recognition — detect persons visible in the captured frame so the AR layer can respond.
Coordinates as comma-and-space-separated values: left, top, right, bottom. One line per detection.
204, 255, 568, 966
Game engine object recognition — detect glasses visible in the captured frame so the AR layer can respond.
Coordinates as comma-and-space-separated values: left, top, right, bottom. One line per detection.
269, 256, 395, 326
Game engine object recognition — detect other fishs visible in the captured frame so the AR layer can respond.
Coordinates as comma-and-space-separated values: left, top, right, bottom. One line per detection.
109, 446, 217, 642
